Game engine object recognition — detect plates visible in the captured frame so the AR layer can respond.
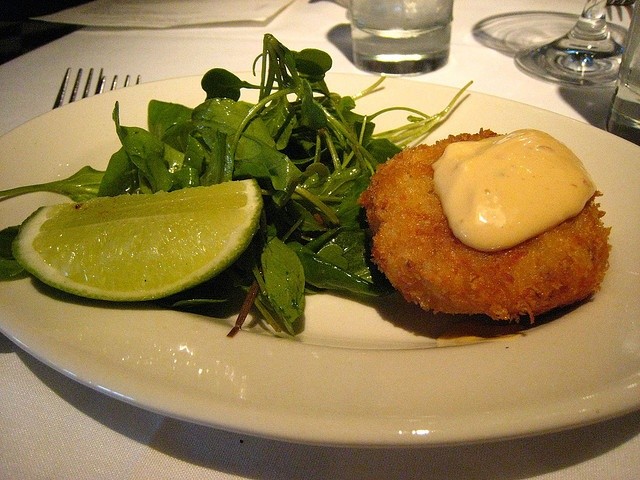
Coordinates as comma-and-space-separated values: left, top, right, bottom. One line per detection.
1, 77, 639, 451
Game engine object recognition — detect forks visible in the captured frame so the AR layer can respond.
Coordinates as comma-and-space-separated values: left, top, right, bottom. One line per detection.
94, 74, 140, 95
52, 67, 104, 109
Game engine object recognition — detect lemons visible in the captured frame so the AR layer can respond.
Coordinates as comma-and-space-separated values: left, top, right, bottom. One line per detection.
11, 177, 265, 306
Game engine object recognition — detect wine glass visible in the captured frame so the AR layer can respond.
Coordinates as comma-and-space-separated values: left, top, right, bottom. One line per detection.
514, 0, 623, 90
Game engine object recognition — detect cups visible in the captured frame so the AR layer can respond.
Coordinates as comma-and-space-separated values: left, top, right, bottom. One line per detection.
605, 0, 640, 146
345, 0, 454, 75
